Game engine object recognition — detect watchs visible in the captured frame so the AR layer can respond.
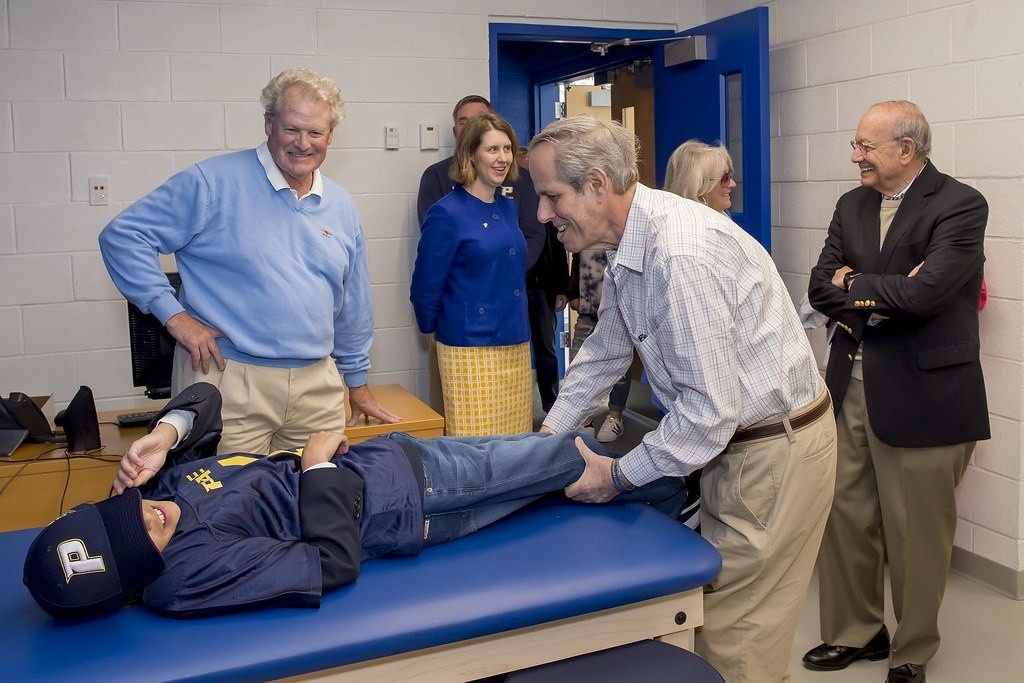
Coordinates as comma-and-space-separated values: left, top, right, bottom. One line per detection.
843, 271, 862, 291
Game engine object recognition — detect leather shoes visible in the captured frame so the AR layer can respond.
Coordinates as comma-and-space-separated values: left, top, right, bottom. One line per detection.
803, 624, 891, 671
886, 663, 927, 683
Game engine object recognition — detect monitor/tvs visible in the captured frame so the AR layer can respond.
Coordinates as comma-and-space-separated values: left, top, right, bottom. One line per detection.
128, 272, 182, 400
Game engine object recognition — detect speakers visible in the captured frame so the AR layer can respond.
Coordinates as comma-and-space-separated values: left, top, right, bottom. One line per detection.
60, 385, 101, 452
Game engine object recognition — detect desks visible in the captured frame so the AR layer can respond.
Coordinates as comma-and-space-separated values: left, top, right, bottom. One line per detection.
1, 381, 447, 533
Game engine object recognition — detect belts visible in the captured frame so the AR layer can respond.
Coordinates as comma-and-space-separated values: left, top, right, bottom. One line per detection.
731, 393, 830, 443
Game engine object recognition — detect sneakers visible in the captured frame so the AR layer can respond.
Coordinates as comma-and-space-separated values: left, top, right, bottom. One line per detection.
678, 471, 701, 532
597, 415, 625, 442
578, 419, 596, 441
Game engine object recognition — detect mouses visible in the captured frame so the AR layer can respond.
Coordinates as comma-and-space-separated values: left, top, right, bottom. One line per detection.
54, 409, 66, 427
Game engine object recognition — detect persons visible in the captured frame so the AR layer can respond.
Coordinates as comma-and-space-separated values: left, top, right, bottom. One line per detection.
528, 118, 838, 682
410, 114, 529, 437
515, 146, 569, 414
418, 95, 545, 270
807, 100, 988, 682
641, 140, 736, 415
98, 67, 402, 458
23, 382, 704, 614
569, 250, 632, 442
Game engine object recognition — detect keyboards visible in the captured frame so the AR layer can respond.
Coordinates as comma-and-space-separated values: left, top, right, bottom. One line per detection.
117, 410, 161, 428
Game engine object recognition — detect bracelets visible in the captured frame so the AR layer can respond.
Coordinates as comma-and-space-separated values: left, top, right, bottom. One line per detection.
612, 458, 635, 495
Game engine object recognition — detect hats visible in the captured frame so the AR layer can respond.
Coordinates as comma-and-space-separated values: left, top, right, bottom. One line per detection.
22, 487, 166, 625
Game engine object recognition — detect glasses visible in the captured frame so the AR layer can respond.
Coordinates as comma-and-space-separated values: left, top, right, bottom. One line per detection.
850, 135, 923, 155
710, 172, 731, 187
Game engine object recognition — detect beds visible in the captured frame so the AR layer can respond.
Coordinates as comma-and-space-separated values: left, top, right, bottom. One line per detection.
0, 497, 723, 683
